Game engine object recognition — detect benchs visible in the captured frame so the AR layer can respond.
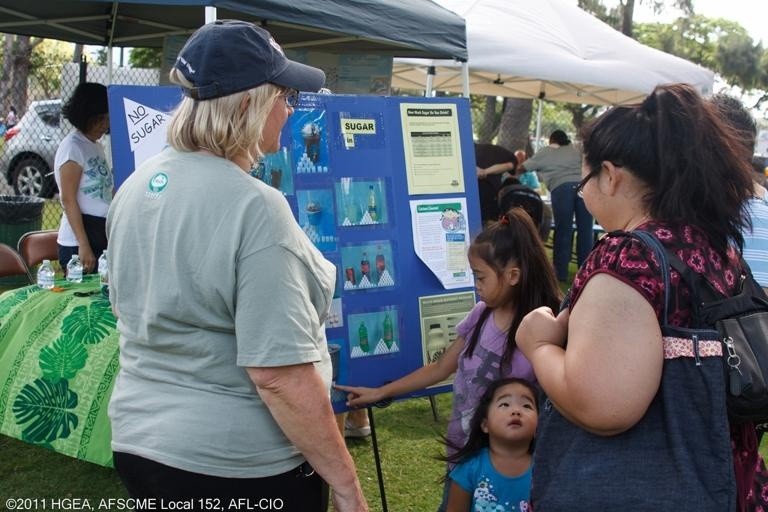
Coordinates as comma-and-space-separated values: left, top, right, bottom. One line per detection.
545, 223, 605, 255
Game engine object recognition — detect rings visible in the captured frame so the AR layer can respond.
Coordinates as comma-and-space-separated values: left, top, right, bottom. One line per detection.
84, 264, 89, 268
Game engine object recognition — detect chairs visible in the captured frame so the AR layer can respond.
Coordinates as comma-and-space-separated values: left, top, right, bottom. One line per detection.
0, 245, 32, 292
18, 230, 57, 285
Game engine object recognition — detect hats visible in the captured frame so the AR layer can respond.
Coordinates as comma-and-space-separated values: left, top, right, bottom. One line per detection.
173, 19, 326, 99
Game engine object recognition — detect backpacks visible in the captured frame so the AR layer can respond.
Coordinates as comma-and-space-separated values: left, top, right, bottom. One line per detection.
668, 253, 767, 422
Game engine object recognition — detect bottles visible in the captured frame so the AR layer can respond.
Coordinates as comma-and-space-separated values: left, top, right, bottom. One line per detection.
383, 315, 393, 348
346, 264, 355, 285
369, 185, 378, 220
66, 254, 83, 283
36, 260, 55, 289
361, 253, 372, 281
376, 245, 386, 281
97, 250, 109, 282
359, 322, 369, 352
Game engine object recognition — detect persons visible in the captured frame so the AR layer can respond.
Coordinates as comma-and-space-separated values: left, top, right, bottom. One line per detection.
516, 83, 768, 512
507, 130, 595, 283
104, 20, 369, 512
52, 82, 115, 280
329, 207, 563, 512
4, 106, 17, 128
701, 92, 768, 453
429, 377, 545, 512
468, 143, 550, 251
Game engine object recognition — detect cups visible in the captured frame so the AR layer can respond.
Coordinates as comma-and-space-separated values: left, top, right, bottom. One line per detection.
305, 208, 322, 226
328, 344, 341, 387
269, 165, 282, 189
253, 163, 265, 179
303, 135, 321, 163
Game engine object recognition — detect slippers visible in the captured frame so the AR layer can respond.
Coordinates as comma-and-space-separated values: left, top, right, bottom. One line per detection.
344, 416, 371, 437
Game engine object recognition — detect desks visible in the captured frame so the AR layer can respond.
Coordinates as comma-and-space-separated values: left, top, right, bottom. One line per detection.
0, 275, 117, 468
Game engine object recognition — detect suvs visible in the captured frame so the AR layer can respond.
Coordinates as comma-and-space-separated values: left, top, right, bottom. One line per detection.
1, 97, 62, 201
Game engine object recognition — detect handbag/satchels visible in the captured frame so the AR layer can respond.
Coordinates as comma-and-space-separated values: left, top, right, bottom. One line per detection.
527, 229, 738, 512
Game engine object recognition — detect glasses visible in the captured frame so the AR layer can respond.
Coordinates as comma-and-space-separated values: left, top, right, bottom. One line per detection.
575, 166, 599, 198
278, 90, 298, 108
